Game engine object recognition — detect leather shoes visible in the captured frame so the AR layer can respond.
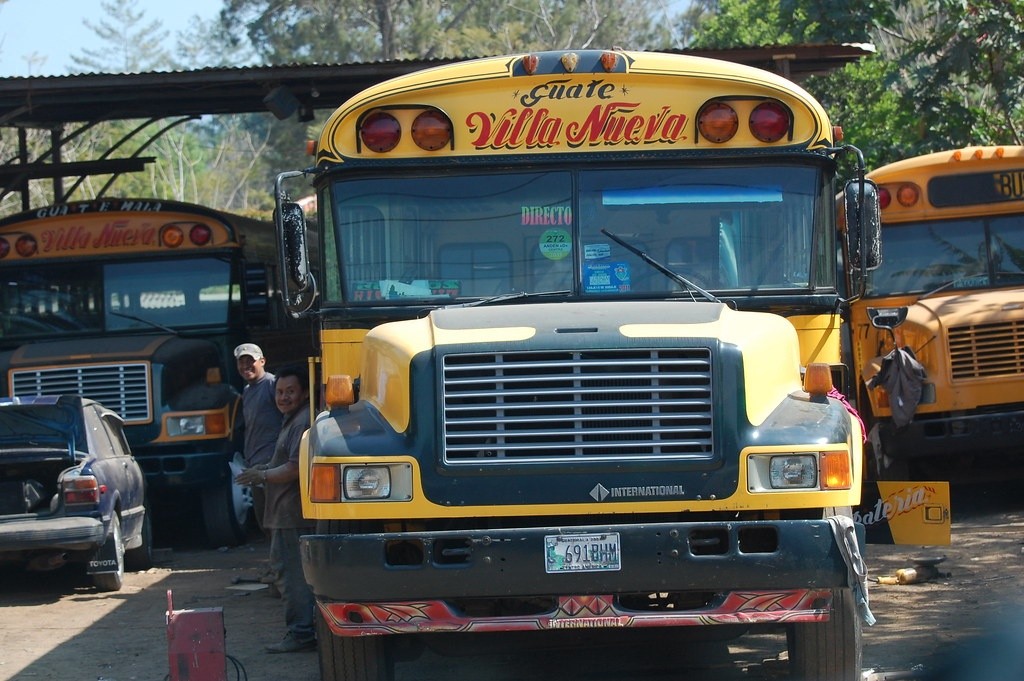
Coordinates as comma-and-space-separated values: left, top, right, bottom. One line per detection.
264, 630, 315, 651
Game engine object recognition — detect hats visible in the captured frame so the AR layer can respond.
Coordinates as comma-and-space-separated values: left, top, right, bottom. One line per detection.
234, 343, 264, 360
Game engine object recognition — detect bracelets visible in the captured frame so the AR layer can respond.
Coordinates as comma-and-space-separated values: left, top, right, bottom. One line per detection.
263, 470, 268, 485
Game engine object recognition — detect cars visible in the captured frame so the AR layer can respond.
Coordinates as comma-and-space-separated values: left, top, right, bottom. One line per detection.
0, 393, 154, 589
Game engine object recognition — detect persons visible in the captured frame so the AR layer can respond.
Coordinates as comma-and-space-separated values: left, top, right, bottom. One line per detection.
234, 364, 316, 654
234, 343, 284, 542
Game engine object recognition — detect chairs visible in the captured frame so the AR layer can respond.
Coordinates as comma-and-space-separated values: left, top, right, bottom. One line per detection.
529, 238, 650, 295
664, 236, 720, 291
436, 240, 514, 298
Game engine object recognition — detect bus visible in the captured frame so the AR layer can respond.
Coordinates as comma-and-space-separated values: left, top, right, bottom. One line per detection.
0, 196, 315, 547
272, 46, 890, 681
835, 145, 1024, 503
